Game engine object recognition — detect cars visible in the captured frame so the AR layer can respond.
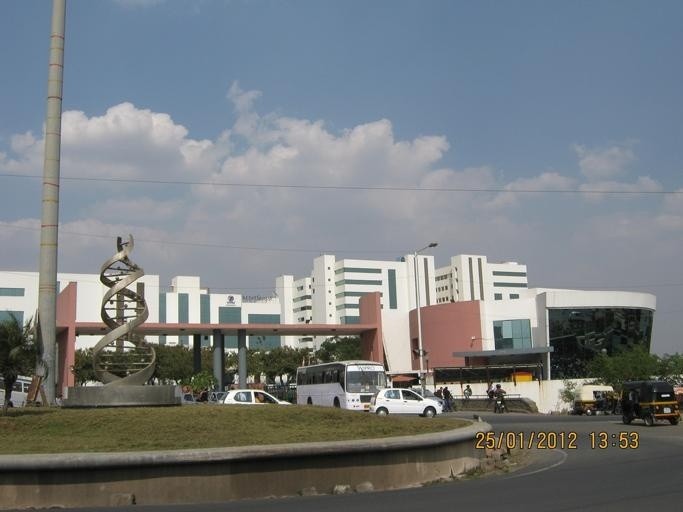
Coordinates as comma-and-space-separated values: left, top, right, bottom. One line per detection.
412, 388, 445, 412
621, 380, 680, 427
218, 389, 291, 405
368, 388, 442, 418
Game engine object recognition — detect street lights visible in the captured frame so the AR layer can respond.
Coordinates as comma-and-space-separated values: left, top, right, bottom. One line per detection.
413, 241, 437, 387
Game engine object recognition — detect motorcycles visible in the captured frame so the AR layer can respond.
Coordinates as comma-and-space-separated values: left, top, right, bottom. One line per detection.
494, 398, 504, 414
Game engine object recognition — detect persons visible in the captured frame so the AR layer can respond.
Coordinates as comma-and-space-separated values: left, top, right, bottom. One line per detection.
485, 381, 496, 398
437, 386, 456, 412
3, 359, 18, 406
204, 379, 214, 391
494, 384, 509, 413
464, 385, 472, 399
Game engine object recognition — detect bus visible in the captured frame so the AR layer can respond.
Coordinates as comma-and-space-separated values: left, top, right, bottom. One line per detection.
296, 359, 391, 413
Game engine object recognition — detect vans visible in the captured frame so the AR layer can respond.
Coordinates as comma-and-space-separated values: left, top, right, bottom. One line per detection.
573, 385, 613, 416
0, 375, 42, 408
